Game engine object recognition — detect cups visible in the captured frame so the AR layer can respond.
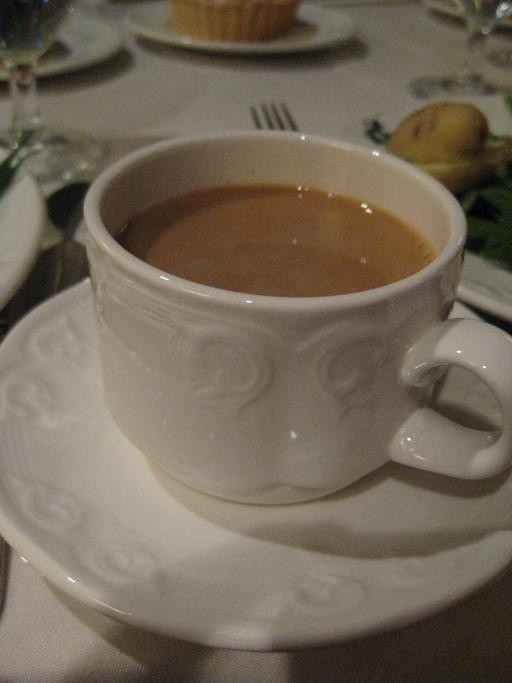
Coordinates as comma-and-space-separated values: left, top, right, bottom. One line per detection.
84, 130, 512, 507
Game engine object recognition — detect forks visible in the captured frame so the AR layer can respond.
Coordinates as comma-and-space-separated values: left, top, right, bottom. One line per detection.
248, 102, 299, 132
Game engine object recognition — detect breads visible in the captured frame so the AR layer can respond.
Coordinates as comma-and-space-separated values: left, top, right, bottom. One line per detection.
168, 0, 303, 42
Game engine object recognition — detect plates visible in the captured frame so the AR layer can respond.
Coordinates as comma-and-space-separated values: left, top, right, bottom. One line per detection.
0, 276, 512, 652
120, 0, 356, 59
346, 94, 511, 325
1, 12, 126, 83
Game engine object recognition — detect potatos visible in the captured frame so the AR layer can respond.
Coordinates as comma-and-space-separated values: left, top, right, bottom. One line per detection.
387, 101, 488, 165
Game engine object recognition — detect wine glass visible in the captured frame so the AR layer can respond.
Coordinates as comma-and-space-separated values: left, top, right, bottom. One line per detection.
0, 0, 109, 185
405, 0, 512, 100
